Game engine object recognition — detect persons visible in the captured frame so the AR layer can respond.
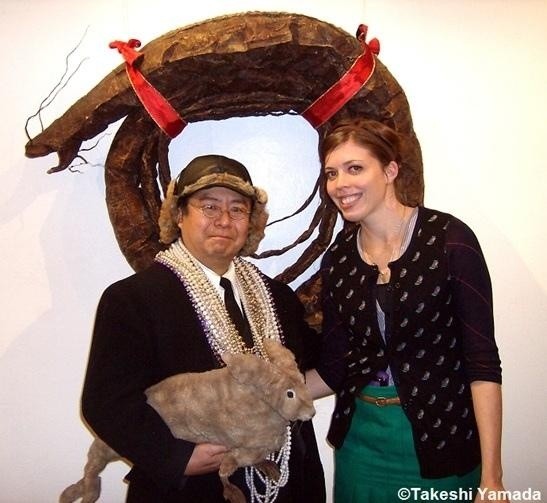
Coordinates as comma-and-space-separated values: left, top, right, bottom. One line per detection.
81, 155, 326, 503
303, 120, 511, 503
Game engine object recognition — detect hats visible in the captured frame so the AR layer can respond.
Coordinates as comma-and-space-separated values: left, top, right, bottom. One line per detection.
157, 155, 267, 257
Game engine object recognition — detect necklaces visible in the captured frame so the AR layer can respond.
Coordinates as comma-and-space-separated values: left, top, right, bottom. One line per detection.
153, 240, 292, 503
362, 204, 407, 282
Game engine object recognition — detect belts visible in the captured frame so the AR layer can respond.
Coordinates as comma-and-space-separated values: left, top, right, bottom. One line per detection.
358, 393, 401, 408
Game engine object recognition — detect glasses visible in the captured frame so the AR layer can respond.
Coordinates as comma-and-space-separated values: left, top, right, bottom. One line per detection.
188, 201, 254, 222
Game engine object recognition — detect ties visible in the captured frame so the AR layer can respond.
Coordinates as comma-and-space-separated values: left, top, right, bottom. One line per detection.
220, 276, 254, 351
